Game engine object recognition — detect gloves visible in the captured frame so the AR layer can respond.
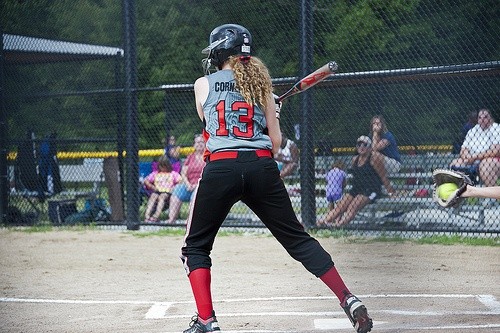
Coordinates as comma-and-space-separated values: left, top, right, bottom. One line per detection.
271, 92, 282, 120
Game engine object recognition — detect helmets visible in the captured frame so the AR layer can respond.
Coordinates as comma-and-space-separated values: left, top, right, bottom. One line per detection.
200, 24, 252, 67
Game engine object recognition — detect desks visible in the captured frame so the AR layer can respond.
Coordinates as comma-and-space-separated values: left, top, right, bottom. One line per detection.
23, 194, 46, 214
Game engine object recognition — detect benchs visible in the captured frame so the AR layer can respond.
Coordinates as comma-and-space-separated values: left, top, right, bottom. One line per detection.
143, 157, 500, 228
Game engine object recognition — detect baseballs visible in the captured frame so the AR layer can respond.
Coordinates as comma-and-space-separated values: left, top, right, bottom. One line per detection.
438, 183, 458, 200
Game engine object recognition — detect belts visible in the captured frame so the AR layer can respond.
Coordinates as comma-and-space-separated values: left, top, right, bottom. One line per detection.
205, 150, 273, 161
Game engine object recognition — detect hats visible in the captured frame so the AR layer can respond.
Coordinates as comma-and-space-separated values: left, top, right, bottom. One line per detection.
357, 134, 372, 144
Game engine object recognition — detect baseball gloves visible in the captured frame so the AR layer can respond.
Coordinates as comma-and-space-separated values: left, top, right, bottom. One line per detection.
433, 169, 474, 208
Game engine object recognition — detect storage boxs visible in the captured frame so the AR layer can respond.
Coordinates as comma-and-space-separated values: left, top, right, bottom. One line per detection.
47, 198, 77, 224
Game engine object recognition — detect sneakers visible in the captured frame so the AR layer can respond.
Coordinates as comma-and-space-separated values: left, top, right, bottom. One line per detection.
182, 310, 221, 333
340, 290, 373, 333
143, 217, 160, 223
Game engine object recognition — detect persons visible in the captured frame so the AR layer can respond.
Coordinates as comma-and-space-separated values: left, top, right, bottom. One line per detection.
274, 132, 299, 181
367, 115, 403, 174
315, 135, 398, 229
179, 22, 373, 333
325, 159, 347, 224
447, 105, 500, 209
142, 129, 207, 225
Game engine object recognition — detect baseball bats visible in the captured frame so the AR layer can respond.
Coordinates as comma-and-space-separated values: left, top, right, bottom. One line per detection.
275, 61, 338, 104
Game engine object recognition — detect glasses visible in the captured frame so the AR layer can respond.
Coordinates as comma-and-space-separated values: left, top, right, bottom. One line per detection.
355, 142, 370, 149
